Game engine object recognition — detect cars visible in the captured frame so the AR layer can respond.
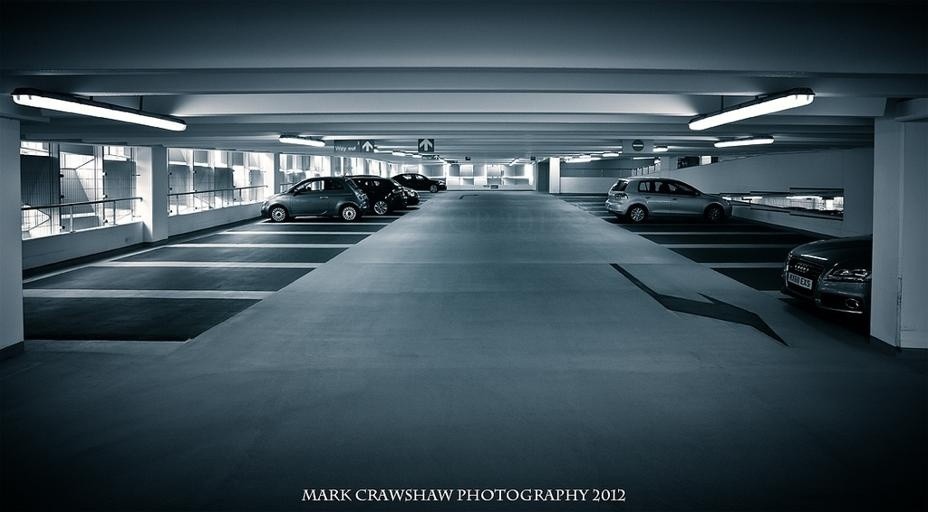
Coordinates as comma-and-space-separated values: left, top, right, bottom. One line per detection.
602, 175, 733, 226
391, 172, 448, 194
778, 231, 872, 318
343, 173, 422, 208
342, 176, 409, 216
260, 175, 370, 223
